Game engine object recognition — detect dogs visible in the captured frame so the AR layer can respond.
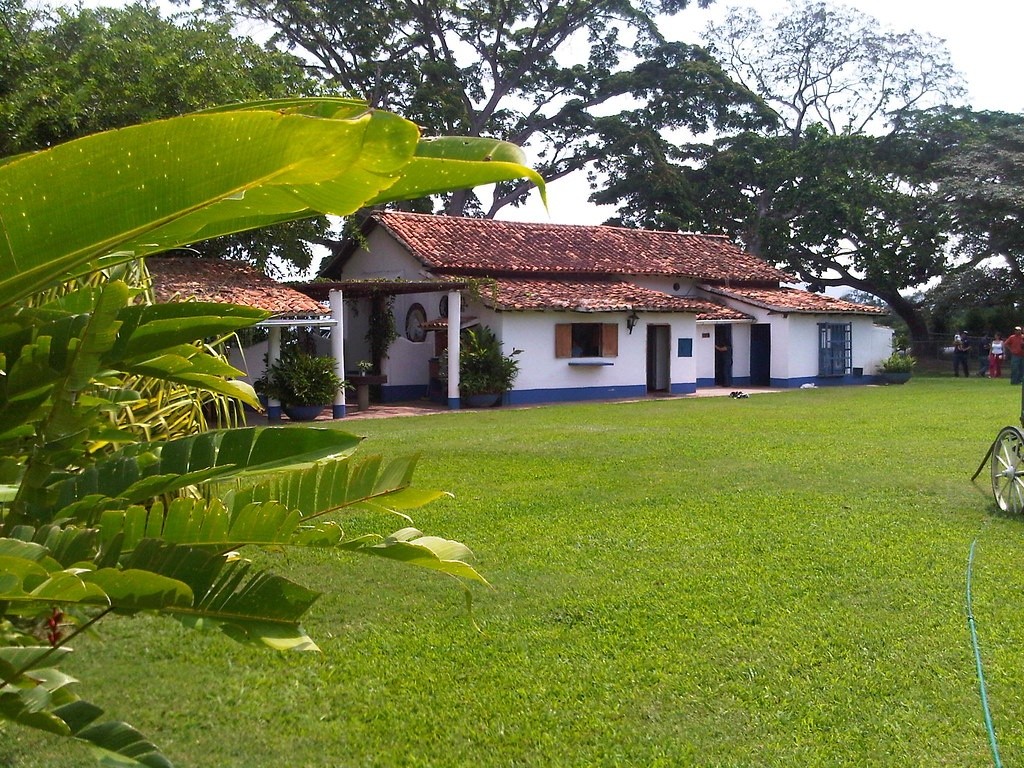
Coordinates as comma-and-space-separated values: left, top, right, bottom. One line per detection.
729, 391, 749, 399
800, 382, 818, 390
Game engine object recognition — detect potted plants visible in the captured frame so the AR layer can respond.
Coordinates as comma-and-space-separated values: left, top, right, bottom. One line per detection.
436, 324, 525, 407
252, 350, 357, 423
357, 360, 374, 376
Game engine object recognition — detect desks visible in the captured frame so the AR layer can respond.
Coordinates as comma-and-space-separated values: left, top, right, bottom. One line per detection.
346, 374, 388, 410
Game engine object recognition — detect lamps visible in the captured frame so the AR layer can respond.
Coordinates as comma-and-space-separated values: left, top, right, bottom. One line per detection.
627, 312, 640, 334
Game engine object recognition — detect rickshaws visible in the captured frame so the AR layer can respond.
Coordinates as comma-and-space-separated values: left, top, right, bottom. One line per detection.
971, 417, 1024, 516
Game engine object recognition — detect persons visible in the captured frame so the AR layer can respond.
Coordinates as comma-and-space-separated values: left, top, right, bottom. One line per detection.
988, 332, 1006, 378
975, 331, 991, 378
1004, 326, 1024, 385
952, 330, 970, 377
953, 330, 963, 351
715, 339, 733, 388
